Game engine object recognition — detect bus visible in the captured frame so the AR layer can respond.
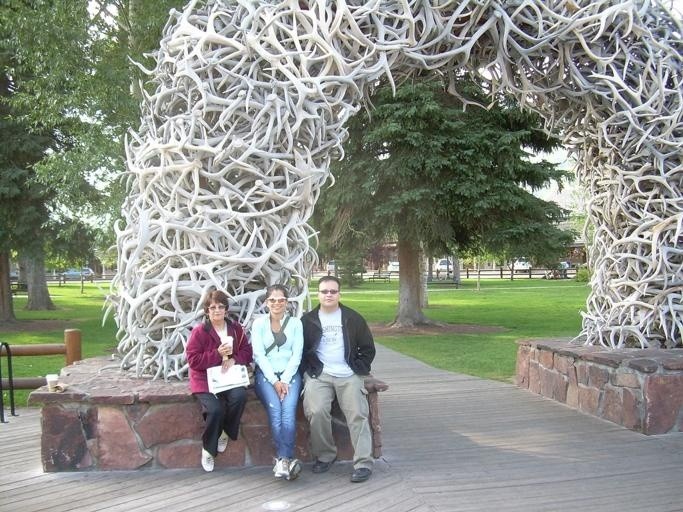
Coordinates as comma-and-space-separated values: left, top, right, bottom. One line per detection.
385, 244, 400, 275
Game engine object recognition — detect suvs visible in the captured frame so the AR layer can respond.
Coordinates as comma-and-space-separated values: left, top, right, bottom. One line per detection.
58, 267, 94, 282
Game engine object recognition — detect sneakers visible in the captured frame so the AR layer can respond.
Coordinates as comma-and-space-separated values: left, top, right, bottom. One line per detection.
273, 458, 302, 481
201, 430, 229, 472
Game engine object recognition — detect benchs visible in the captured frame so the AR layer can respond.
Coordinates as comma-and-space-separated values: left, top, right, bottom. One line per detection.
368, 272, 391, 283
9, 282, 27, 296
425, 274, 459, 290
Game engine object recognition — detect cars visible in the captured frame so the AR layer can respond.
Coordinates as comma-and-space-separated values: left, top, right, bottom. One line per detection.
509, 256, 533, 274
326, 260, 339, 272
436, 258, 452, 272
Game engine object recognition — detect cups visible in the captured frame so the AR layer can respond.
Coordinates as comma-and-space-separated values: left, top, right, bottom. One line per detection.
45, 374, 58, 391
221, 336, 233, 355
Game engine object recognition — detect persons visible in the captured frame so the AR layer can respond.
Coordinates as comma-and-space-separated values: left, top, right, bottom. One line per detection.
250, 284, 304, 482
186, 290, 253, 473
299, 275, 377, 483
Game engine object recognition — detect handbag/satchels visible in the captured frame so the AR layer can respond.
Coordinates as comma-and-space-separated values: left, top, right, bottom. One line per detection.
247, 363, 256, 388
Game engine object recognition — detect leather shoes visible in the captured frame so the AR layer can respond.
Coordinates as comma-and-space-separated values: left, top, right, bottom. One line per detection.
351, 468, 371, 482
311, 457, 336, 473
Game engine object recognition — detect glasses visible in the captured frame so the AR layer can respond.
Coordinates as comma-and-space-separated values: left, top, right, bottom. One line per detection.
268, 297, 286, 303
209, 305, 226, 310
320, 290, 338, 294
